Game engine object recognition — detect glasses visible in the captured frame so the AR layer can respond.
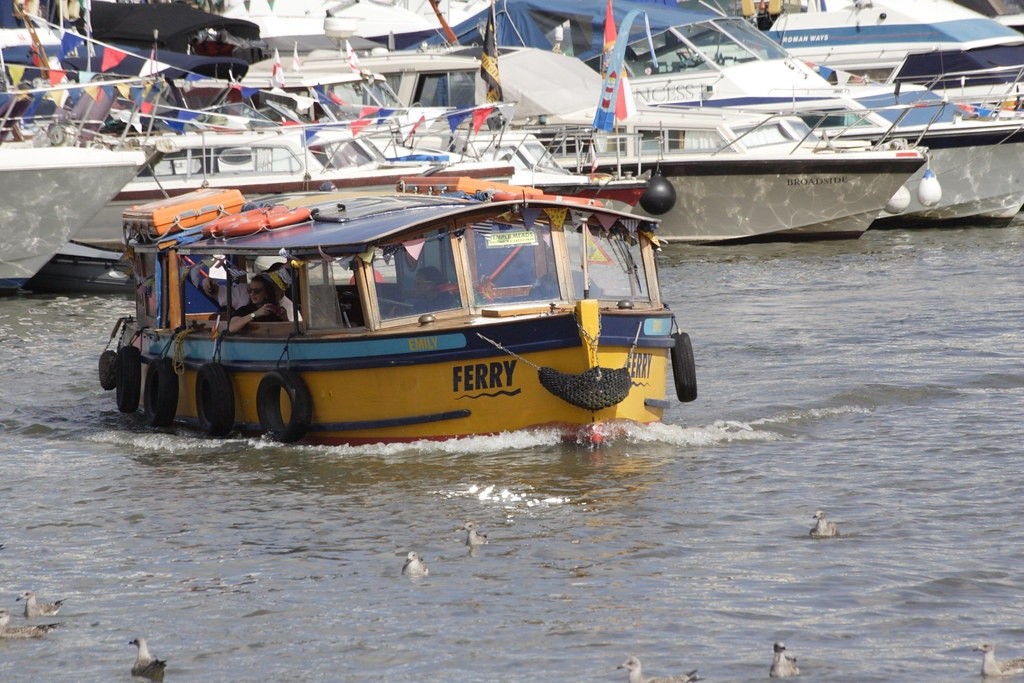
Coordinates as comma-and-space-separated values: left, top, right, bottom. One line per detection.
247, 286, 264, 294
413, 278, 432, 286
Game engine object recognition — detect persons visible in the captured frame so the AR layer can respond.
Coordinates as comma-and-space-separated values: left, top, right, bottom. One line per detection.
228, 275, 290, 334
414, 266, 458, 314
202, 262, 303, 323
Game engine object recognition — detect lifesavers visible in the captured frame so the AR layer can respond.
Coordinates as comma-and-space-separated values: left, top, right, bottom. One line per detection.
203, 204, 309, 238
492, 190, 605, 208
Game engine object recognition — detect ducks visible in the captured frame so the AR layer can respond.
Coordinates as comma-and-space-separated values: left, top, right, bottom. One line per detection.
401, 551, 429, 576
128, 637, 167, 683
809, 511, 840, 536
770, 641, 800, 678
16, 591, 68, 618
461, 522, 488, 545
972, 642, 1024, 677
617, 656, 703, 683
0, 608, 61, 637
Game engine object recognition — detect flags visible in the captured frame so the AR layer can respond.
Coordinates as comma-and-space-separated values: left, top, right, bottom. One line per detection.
604, 0, 631, 123
480, 1, 505, 119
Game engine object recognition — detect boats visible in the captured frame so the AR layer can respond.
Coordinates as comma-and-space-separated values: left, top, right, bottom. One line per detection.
486, 13, 930, 248
62, 117, 657, 253
762, 0, 1024, 113
98, 168, 701, 447
828, 79, 1024, 224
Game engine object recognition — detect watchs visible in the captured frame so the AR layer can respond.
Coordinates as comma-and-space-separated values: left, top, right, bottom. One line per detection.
250, 312, 256, 320
